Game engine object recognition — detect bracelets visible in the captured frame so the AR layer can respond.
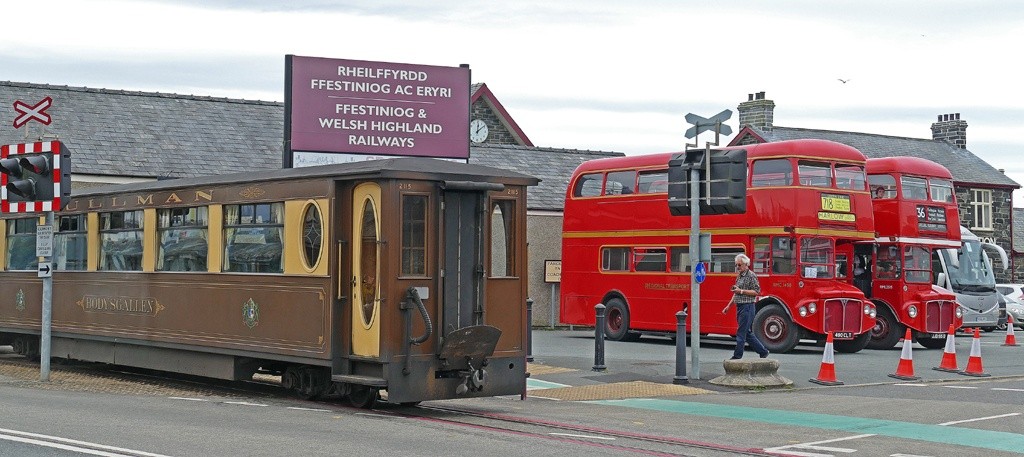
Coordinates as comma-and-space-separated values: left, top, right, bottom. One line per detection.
740, 290, 743, 294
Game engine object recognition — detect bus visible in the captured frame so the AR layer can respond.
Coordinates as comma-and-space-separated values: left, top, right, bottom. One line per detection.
649, 155, 965, 351
559, 138, 877, 355
934, 224, 1008, 333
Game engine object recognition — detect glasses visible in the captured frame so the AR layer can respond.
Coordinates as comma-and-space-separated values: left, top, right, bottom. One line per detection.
735, 264, 745, 266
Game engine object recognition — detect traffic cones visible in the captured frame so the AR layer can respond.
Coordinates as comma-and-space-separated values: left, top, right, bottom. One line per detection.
808, 331, 845, 386
1000, 316, 1021, 347
932, 324, 962, 373
958, 327, 991, 377
887, 327, 921, 381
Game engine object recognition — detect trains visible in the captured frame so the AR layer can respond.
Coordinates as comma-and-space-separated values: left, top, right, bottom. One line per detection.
0, 157, 534, 410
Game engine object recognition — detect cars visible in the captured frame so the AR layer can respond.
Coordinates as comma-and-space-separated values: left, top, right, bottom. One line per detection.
982, 289, 1007, 331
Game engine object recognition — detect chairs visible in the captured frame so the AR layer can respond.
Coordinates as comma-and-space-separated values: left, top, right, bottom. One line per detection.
774, 257, 793, 274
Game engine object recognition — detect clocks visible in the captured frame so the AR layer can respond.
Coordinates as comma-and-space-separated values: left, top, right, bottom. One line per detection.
470, 119, 488, 143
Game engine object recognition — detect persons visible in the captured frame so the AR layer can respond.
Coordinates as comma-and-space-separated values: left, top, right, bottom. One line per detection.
840, 249, 896, 296
722, 254, 770, 360
873, 187, 885, 199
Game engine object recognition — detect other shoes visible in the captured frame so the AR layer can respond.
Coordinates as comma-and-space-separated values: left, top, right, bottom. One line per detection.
729, 357, 742, 360
760, 350, 770, 358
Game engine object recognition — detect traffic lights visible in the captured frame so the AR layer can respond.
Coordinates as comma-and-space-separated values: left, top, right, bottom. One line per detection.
0, 151, 54, 201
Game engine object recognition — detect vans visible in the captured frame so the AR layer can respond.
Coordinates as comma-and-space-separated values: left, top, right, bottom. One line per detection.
996, 283, 1024, 331
